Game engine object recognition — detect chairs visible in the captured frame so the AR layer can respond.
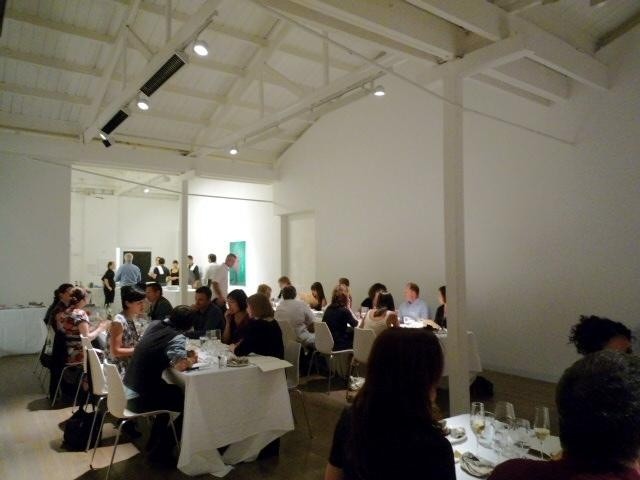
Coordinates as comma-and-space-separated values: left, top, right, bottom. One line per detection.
347, 327, 379, 394
31, 308, 97, 405
90, 361, 181, 479
307, 323, 354, 391
75, 347, 108, 445
278, 340, 313, 443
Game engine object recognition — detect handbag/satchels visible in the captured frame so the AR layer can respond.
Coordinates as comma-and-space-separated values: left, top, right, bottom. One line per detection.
61, 409, 102, 451
40, 353, 58, 370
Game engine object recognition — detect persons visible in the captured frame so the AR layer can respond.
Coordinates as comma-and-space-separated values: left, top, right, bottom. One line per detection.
570, 313, 634, 356
101, 252, 236, 313
484, 346, 640, 479
325, 327, 456, 480
43, 283, 286, 462
274, 276, 447, 351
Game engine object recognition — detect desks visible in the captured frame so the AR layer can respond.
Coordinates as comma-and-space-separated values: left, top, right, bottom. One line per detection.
309, 309, 449, 391
0, 304, 50, 357
76, 307, 288, 471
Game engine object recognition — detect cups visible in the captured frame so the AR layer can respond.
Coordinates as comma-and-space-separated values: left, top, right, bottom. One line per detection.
353, 305, 410, 326
134, 312, 227, 370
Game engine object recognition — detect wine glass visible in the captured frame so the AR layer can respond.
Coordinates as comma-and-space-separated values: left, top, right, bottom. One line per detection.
468, 401, 551, 464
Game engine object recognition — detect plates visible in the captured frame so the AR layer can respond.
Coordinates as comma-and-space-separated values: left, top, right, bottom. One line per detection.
227, 360, 250, 368
446, 429, 468, 443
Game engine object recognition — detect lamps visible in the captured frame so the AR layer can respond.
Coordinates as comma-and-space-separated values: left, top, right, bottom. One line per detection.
228, 144, 242, 157
361, 85, 387, 100
93, 18, 220, 149
